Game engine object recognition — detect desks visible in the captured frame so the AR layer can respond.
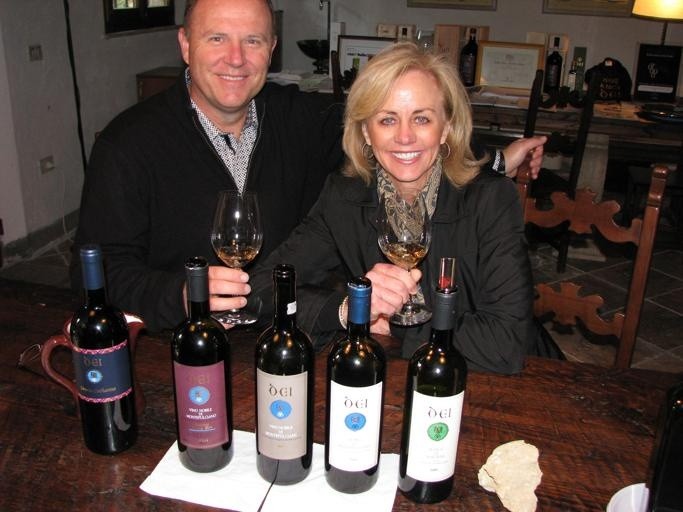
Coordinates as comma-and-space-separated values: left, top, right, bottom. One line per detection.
486, 144, 666, 372
0, 264, 682, 511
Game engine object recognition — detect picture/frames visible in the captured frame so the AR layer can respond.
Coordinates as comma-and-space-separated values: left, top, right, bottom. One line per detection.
543, 0, 633, 18
474, 40, 546, 96
406, 1, 497, 13
337, 35, 394, 83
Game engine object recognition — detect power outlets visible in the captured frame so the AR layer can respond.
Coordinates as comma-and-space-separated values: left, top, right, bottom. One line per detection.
40, 155, 55, 175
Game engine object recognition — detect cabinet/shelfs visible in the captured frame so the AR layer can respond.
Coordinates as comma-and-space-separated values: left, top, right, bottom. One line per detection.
267, 71, 682, 254
136, 65, 186, 102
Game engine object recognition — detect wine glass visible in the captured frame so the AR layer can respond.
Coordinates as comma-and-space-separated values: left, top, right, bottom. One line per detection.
209, 190, 262, 324
375, 190, 432, 328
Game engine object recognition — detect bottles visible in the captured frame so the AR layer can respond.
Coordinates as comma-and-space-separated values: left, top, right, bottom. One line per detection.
545, 35, 565, 92
566, 57, 583, 94
460, 27, 479, 85
169, 253, 234, 475
253, 263, 315, 486
322, 274, 388, 494
69, 246, 139, 456
396, 258, 468, 503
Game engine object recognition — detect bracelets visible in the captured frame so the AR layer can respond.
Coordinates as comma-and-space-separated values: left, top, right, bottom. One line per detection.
498, 152, 505, 174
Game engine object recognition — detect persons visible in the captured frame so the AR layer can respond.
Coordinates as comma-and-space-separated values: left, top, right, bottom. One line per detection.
246, 40, 532, 376
70, 0, 549, 331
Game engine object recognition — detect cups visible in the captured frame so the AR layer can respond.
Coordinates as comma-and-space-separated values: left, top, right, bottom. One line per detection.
41, 312, 146, 414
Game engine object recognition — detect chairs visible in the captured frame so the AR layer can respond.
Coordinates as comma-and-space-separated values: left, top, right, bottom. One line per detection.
478, 78, 599, 272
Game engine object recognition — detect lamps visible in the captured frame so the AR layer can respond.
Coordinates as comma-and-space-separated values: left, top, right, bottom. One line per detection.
630, 0, 683, 47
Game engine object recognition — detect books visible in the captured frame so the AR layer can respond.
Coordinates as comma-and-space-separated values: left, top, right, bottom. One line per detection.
630, 40, 683, 102
